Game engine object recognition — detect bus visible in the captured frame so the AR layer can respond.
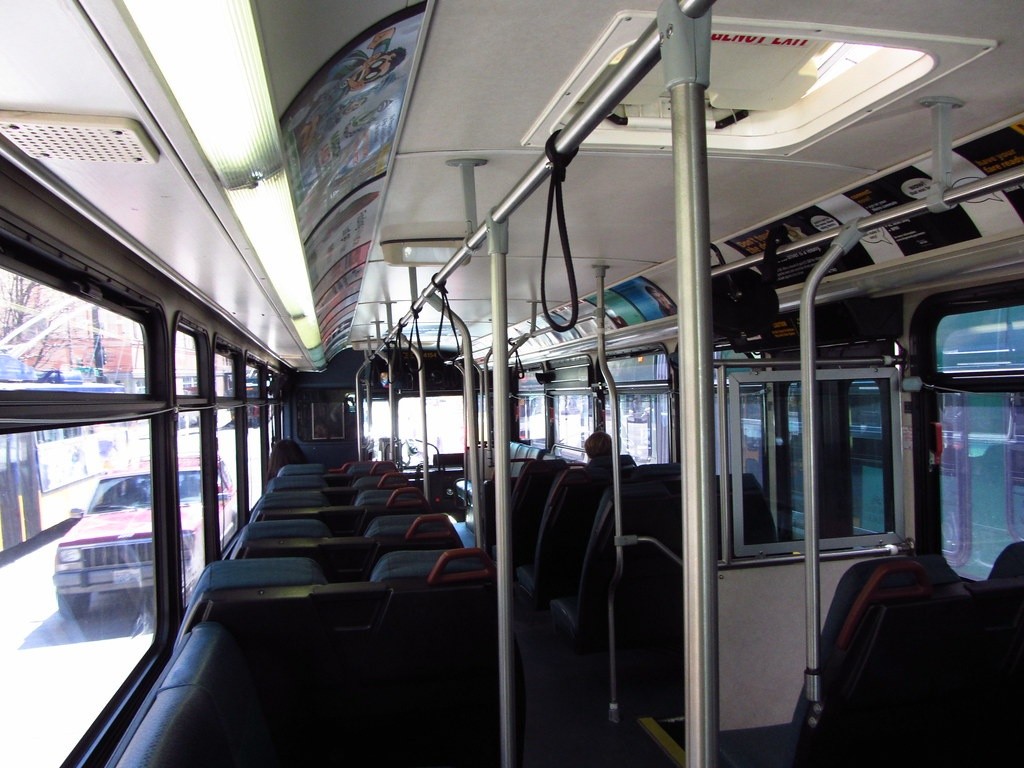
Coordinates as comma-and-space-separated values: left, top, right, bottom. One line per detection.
0, 353, 138, 553
228, 383, 274, 427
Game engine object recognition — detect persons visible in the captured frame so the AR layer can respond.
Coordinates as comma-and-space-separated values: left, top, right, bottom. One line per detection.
583, 431, 612, 470
268, 439, 309, 478
380, 372, 400, 388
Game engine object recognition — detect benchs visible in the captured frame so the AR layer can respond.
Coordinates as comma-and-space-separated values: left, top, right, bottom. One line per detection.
111, 443, 1024, 768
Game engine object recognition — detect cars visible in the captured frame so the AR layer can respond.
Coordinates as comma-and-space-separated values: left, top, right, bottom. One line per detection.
627, 410, 649, 423
179, 411, 199, 429
605, 405, 610, 413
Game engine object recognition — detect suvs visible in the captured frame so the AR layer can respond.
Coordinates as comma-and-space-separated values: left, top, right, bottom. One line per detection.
52, 455, 237, 621
